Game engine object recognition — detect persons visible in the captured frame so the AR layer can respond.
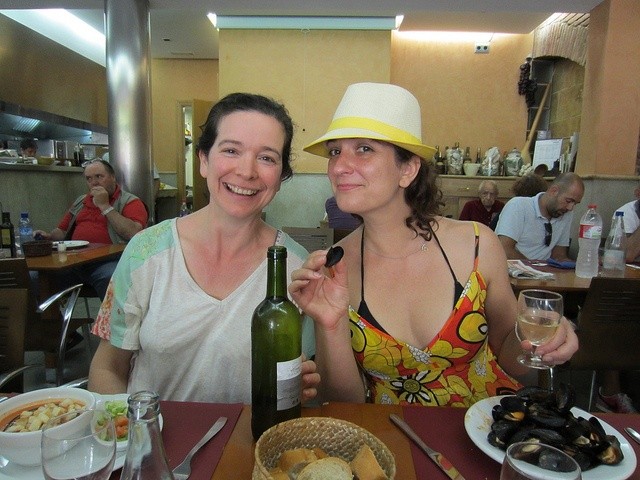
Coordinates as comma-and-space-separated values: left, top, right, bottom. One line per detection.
325, 196, 363, 230
102, 152, 161, 201
609, 186, 640, 265
86, 93, 320, 409
489, 173, 548, 231
33, 158, 149, 351
535, 164, 548, 176
458, 180, 504, 226
494, 172, 585, 261
19, 139, 37, 157
289, 82, 580, 406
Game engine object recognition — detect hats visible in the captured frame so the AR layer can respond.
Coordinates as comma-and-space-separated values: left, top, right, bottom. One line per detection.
303, 82, 438, 163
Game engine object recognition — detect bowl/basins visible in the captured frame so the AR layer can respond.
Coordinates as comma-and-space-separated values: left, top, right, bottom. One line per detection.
21, 239, 53, 258
0, 386, 95, 467
463, 164, 482, 176
36, 156, 55, 164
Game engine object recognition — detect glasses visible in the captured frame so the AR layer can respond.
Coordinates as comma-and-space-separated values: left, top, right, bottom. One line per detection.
544, 220, 552, 246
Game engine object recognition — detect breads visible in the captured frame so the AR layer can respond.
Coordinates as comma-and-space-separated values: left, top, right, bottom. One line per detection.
269, 443, 388, 480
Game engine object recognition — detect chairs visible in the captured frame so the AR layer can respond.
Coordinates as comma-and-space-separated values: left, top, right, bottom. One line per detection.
59, 376, 89, 387
281, 227, 334, 255
0, 259, 95, 387
0, 288, 31, 393
569, 277, 639, 413
320, 221, 355, 244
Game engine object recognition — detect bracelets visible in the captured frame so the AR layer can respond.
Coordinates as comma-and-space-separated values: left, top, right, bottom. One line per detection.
101, 207, 114, 216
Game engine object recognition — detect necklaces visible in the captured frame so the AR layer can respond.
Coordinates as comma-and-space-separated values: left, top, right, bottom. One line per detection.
366, 235, 430, 262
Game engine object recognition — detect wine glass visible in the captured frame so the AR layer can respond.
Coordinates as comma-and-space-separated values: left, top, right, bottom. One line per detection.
40, 409, 117, 480
515, 290, 564, 370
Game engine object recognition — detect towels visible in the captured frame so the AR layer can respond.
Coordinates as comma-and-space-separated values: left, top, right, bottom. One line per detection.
547, 260, 575, 269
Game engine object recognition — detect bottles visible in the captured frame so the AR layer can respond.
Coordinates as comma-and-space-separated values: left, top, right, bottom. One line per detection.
574, 205, 603, 279
18, 212, 35, 247
0, 212, 15, 259
463, 147, 471, 164
119, 390, 175, 480
251, 245, 303, 441
436, 152, 444, 174
603, 209, 627, 278
475, 146, 483, 164
499, 152, 507, 175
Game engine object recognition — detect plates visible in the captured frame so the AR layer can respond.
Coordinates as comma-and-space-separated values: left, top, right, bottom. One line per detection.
52, 240, 90, 249
1, 156, 22, 163
464, 395, 637, 479
0, 393, 165, 480
90, 394, 131, 448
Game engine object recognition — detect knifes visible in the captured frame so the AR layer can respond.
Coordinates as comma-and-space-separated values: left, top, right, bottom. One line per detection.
386, 411, 464, 479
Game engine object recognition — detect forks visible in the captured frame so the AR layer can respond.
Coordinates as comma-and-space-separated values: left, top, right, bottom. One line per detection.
172, 415, 229, 479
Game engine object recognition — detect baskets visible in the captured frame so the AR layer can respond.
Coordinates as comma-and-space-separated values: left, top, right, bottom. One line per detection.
251, 416, 396, 479
